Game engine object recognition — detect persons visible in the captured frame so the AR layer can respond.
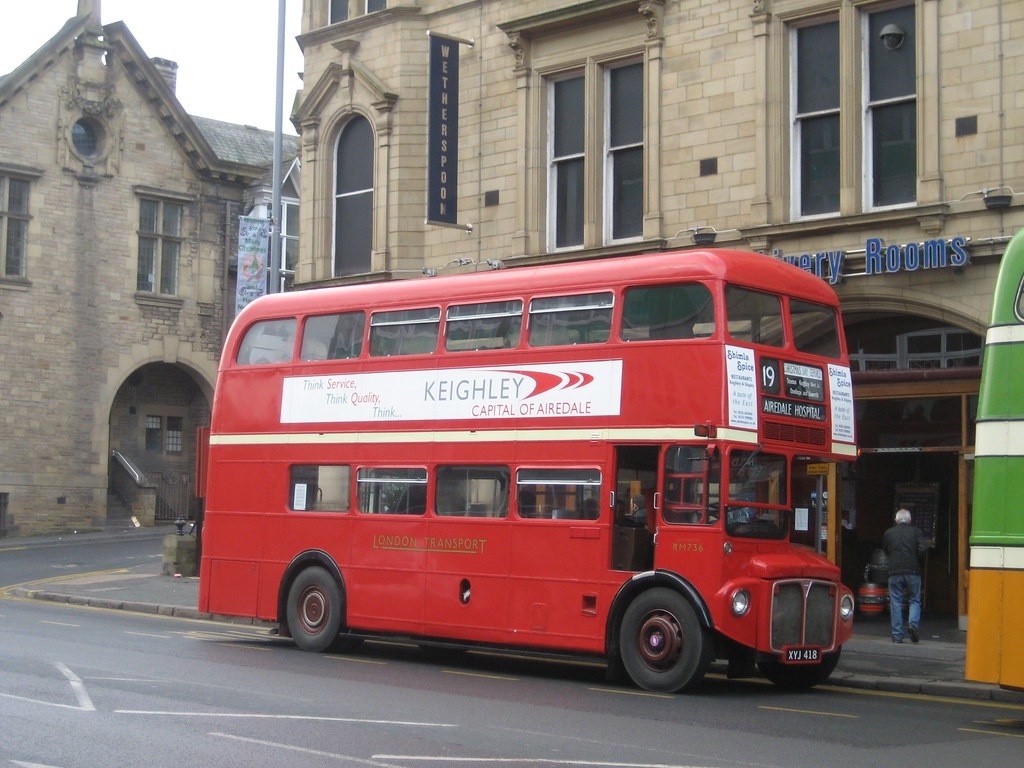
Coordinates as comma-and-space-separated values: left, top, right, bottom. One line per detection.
630, 493, 646, 515
883, 508, 929, 643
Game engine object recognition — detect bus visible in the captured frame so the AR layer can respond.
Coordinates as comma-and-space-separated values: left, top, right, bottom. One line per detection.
198, 247, 860, 696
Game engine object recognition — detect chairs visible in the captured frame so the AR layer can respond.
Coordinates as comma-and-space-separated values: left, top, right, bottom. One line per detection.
298, 477, 749, 571
273, 312, 695, 360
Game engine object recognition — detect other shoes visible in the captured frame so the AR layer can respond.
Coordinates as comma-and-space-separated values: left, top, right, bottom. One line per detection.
907, 625, 919, 642
893, 640, 902, 643
